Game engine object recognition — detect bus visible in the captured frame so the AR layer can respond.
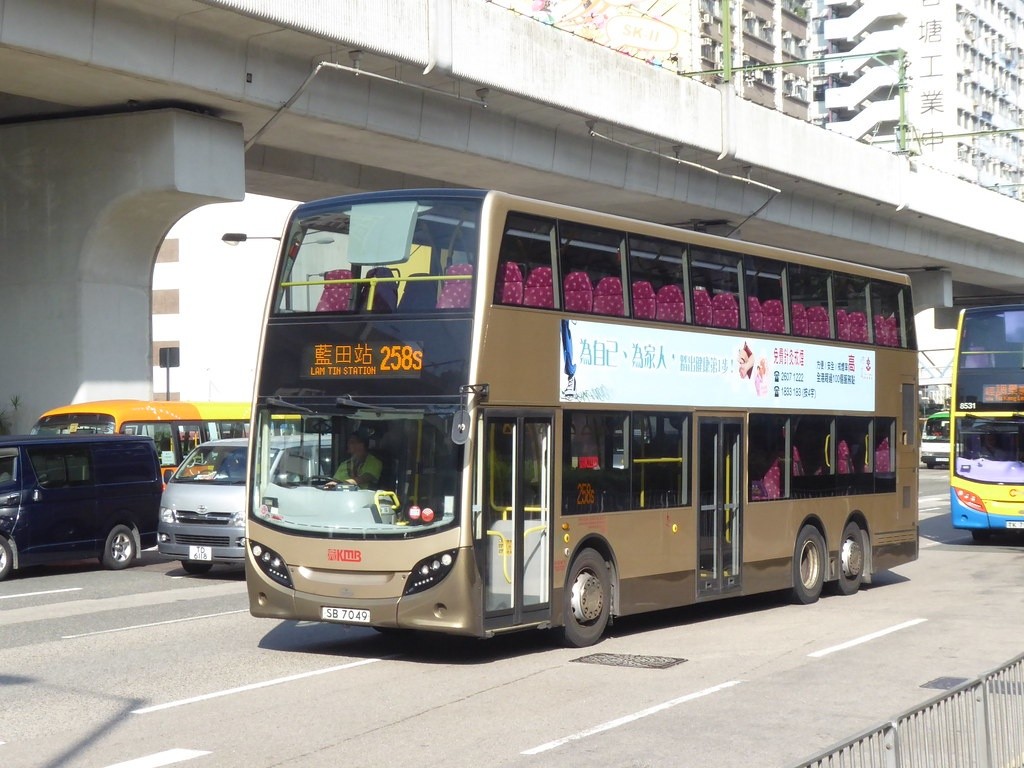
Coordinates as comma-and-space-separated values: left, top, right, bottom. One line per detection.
244, 185, 920, 647
29, 399, 301, 493
950, 304, 1024, 543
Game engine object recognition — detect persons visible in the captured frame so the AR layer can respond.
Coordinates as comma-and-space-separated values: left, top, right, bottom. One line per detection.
323, 430, 382, 491
930, 426, 943, 436
980, 431, 1016, 461
213, 452, 246, 483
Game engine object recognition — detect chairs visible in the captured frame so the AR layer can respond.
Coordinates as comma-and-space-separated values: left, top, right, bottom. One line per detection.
375, 431, 402, 490
313, 263, 904, 351
746, 435, 893, 501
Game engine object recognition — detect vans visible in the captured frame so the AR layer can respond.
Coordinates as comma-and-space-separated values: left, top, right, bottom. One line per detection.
0, 435, 161, 579
157, 433, 335, 573
920, 412, 950, 468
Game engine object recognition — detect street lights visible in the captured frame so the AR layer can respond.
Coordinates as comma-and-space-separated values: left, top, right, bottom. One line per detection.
220, 232, 334, 311
